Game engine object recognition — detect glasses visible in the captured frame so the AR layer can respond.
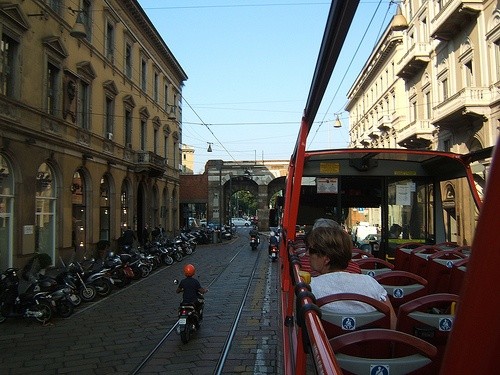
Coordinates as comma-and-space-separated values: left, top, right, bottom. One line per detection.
308, 247, 316, 254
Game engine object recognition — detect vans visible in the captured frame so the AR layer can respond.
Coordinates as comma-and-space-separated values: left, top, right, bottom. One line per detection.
229, 218, 252, 227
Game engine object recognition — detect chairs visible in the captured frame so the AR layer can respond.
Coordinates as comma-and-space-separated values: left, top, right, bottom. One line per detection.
293, 237, 475, 375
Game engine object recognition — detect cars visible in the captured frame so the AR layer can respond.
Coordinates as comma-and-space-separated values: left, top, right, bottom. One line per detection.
199, 219, 207, 227
188, 217, 195, 226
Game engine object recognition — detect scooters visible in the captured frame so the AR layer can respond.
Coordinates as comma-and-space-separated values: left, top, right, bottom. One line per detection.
267, 237, 279, 261
173, 279, 208, 344
138, 225, 234, 265
249, 232, 262, 250
0, 243, 160, 327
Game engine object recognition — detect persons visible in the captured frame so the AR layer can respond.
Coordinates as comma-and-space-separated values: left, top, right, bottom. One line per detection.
305, 227, 397, 330
299, 218, 359, 276
249, 227, 260, 240
268, 231, 279, 255
122, 226, 136, 248
388, 224, 402, 239
143, 224, 164, 244
176, 264, 208, 314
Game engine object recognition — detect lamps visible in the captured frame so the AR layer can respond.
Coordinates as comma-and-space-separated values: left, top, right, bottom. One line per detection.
168, 106, 176, 119
71, 11, 87, 38
334, 119, 342, 127
207, 144, 212, 152
391, 3, 409, 31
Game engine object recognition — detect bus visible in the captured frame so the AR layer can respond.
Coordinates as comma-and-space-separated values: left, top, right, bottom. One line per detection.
269, 0, 500, 375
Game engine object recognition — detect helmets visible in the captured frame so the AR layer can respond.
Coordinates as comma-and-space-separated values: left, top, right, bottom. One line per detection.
271, 232, 274, 236
184, 265, 195, 276
123, 245, 131, 251
108, 252, 116, 257
